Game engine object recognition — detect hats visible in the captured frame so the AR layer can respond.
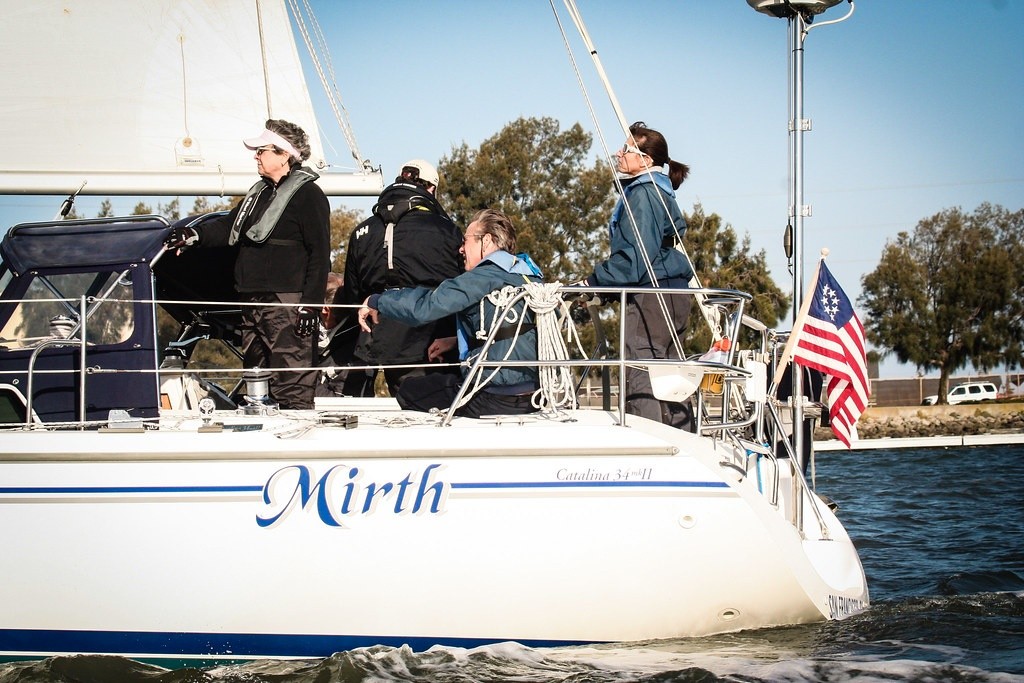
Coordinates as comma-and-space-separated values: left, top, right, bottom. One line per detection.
243, 129, 304, 163
399, 160, 439, 199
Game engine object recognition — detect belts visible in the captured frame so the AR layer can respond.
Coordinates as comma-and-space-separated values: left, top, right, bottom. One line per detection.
490, 393, 541, 402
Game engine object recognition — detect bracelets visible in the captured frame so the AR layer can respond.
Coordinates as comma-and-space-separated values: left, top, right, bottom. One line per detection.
583, 279, 589, 287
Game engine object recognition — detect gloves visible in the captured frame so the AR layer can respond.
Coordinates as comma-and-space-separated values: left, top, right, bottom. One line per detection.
296, 307, 319, 335
582, 294, 607, 308
161, 227, 199, 256
562, 280, 595, 303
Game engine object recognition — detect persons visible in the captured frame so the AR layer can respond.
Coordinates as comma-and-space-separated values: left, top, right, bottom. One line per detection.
319, 153, 561, 420
561, 121, 696, 434
161, 118, 332, 411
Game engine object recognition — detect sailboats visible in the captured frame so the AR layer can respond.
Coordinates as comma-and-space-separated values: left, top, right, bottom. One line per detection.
0, 0, 869, 660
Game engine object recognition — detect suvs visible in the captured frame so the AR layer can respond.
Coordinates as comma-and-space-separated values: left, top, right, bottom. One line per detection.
922, 381, 998, 406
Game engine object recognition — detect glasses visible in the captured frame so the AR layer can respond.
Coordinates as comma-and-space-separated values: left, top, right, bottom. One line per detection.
256, 147, 276, 154
622, 143, 653, 162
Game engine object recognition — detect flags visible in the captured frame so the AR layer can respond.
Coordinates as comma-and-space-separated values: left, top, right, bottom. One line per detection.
783, 256, 872, 454
